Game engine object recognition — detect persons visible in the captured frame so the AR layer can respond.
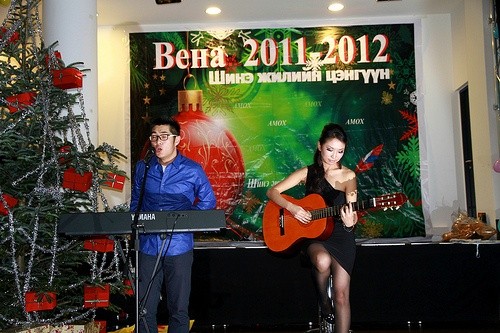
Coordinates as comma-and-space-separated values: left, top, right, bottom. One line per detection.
128, 120, 217, 333
267, 123, 359, 333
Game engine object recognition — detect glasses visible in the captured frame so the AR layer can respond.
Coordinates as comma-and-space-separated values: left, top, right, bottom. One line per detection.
149, 134, 177, 142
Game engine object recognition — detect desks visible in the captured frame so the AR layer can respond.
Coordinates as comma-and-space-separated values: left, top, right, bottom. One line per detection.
193, 236, 500, 333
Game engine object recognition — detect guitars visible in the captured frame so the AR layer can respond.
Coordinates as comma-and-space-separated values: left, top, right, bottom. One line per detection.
262, 193, 407, 252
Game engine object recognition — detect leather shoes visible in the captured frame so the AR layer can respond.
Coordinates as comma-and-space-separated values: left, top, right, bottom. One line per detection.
319, 297, 336, 324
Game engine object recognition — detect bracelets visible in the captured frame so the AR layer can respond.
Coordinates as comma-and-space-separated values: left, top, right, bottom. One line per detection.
343, 223, 355, 233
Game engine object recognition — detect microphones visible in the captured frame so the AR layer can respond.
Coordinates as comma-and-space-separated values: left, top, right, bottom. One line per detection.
142, 147, 155, 165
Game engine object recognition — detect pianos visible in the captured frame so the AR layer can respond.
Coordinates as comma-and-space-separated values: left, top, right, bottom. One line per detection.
57, 208, 226, 333
